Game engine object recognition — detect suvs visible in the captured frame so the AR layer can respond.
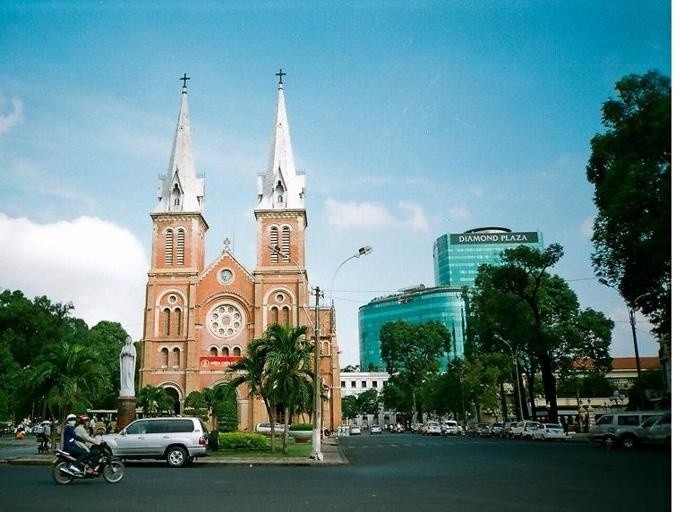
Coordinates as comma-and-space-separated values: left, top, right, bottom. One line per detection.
613, 413, 671, 451
254, 422, 288, 434
586, 414, 613, 444
90, 416, 209, 468
0, 421, 16, 434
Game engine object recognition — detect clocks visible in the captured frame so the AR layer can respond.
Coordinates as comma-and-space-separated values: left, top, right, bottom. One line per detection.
218, 269, 232, 281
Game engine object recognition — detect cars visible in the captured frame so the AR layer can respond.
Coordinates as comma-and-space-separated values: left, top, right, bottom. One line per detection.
34, 420, 52, 435
466, 419, 572, 443
367, 425, 383, 434
385, 422, 463, 435
350, 426, 361, 435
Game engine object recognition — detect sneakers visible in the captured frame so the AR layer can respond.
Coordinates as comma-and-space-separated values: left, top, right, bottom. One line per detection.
69, 465, 80, 472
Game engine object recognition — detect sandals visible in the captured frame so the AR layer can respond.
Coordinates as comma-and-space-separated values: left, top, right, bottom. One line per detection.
85, 468, 99, 474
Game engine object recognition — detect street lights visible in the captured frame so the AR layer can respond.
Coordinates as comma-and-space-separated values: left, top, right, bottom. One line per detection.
609, 390, 625, 412
595, 275, 659, 383
263, 239, 375, 464
320, 348, 344, 434
442, 357, 470, 424
599, 400, 613, 415
490, 335, 526, 421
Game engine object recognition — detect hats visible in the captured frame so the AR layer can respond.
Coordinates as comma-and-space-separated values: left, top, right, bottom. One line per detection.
66, 414, 76, 420
79, 415, 89, 421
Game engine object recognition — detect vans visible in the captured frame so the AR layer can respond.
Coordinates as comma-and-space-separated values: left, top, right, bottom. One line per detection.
608, 413, 663, 441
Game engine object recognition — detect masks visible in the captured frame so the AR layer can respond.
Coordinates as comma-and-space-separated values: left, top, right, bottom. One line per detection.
67, 420, 76, 426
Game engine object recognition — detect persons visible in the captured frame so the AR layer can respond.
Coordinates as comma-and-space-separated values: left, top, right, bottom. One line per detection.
17, 413, 117, 476
119, 335, 137, 391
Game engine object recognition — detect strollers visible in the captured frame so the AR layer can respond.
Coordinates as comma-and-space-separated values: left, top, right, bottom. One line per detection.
15, 427, 26, 440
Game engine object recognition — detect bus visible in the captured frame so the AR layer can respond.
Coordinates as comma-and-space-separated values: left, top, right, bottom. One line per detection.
83, 408, 175, 437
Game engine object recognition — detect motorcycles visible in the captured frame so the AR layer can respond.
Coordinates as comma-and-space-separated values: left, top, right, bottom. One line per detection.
51, 432, 127, 486
33, 427, 54, 454
